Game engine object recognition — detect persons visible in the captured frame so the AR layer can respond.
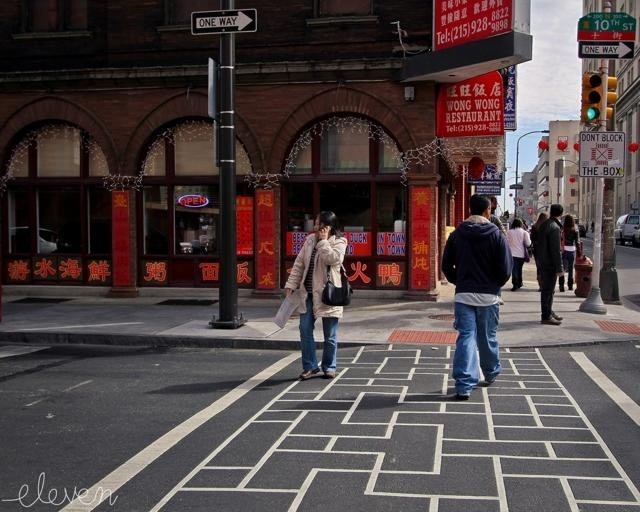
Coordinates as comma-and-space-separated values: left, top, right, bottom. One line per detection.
534, 205, 564, 325
284, 209, 352, 378
504, 218, 532, 290
530, 211, 548, 292
443, 195, 513, 399
489, 208, 506, 305
558, 214, 579, 291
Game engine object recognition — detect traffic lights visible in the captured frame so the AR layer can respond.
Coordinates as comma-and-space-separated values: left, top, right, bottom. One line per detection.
580, 70, 605, 121
606, 77, 617, 119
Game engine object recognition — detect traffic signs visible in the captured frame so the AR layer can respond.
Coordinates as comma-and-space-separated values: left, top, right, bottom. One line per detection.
190, 9, 258, 34
581, 43, 635, 60
578, 14, 637, 40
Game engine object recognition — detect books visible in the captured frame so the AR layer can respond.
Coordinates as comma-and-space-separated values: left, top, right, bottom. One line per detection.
275, 292, 297, 330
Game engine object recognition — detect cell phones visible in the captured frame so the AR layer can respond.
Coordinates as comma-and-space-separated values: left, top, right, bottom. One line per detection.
324, 225, 333, 232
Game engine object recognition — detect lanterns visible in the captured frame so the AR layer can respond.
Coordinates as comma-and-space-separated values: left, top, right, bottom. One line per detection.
538, 140, 547, 150
628, 143, 639, 152
556, 140, 568, 151
573, 143, 582, 150
468, 156, 486, 179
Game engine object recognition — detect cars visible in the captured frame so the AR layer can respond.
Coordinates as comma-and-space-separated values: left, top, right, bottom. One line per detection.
145, 228, 193, 254
8, 226, 68, 254
631, 225, 640, 248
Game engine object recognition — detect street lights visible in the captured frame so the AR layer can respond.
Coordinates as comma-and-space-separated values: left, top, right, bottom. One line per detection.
503, 176, 520, 212
514, 129, 550, 216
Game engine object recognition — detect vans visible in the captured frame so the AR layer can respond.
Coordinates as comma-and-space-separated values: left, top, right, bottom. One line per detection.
615, 214, 640, 244
577, 225, 587, 238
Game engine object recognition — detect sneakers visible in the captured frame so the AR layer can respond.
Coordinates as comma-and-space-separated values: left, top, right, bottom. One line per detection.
300, 367, 320, 380
323, 368, 336, 379
542, 310, 563, 325
455, 389, 470, 400
483, 362, 502, 384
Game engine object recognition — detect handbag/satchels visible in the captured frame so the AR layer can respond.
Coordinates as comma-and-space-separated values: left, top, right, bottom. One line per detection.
322, 274, 352, 306
524, 245, 530, 262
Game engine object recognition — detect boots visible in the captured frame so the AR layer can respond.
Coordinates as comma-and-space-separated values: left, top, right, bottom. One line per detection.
559, 275, 573, 292
